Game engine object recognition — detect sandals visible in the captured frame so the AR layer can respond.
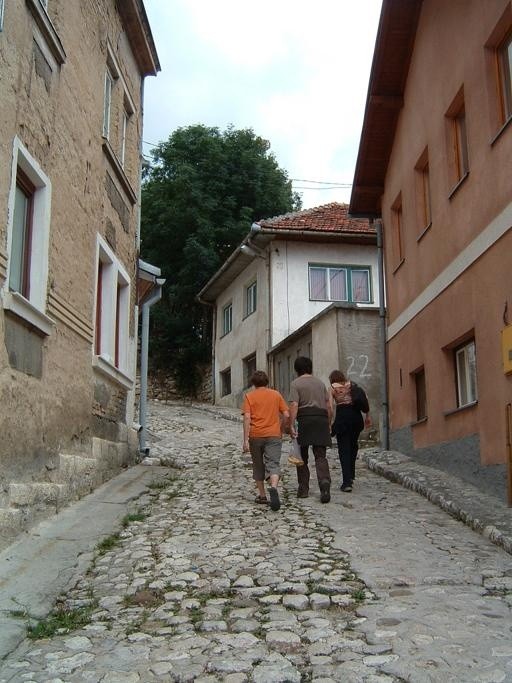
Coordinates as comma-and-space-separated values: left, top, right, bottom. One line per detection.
255, 487, 280, 511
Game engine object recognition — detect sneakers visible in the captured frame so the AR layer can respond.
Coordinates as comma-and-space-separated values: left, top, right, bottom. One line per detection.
320, 479, 330, 503
297, 490, 308, 498
341, 481, 353, 491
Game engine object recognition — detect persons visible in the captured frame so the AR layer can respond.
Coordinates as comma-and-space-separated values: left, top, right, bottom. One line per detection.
324, 368, 372, 492
286, 355, 333, 503
239, 367, 290, 511
